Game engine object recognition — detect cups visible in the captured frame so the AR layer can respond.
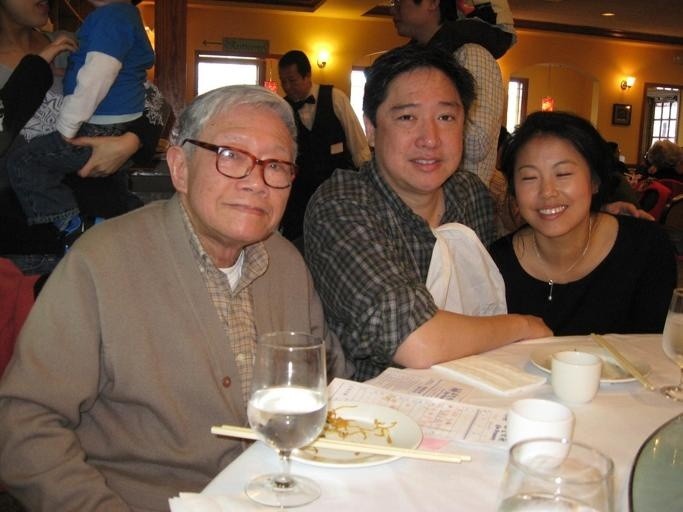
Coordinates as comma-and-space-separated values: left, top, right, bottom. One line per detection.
495, 398, 615, 512
551, 350, 602, 407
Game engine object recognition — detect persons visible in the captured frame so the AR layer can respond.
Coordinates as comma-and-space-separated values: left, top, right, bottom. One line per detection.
1, 1, 172, 272
390, 1, 518, 238
280, 50, 374, 262
597, 140, 681, 222
305, 46, 656, 380
485, 109, 676, 335
1, 85, 354, 512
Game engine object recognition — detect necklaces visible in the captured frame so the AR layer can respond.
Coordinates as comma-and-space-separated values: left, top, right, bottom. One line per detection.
533, 217, 592, 300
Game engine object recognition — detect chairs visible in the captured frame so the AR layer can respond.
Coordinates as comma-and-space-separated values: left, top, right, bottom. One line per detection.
658, 194, 683, 226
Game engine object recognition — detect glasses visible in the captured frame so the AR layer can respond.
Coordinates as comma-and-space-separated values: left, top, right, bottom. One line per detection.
180, 139, 298, 188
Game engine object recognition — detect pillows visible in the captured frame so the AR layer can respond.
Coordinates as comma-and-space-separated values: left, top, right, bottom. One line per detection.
637, 181, 672, 221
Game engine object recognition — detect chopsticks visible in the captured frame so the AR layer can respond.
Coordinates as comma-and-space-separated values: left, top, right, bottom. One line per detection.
590, 332, 654, 391
211, 424, 472, 464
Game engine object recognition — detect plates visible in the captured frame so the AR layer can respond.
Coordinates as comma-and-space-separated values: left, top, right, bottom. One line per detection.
627, 412, 683, 512
297, 401, 423, 467
529, 335, 654, 385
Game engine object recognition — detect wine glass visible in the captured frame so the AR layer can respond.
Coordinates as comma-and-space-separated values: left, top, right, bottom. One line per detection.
245, 333, 328, 508
661, 288, 683, 404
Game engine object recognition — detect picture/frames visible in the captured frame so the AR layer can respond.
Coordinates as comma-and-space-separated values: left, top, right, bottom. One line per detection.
612, 104, 632, 125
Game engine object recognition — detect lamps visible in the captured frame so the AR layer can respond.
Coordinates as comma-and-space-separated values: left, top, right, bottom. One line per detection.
621, 77, 636, 89
541, 65, 554, 112
317, 50, 329, 68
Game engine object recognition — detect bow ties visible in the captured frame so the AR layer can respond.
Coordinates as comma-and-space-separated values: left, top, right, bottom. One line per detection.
294, 94, 316, 110
